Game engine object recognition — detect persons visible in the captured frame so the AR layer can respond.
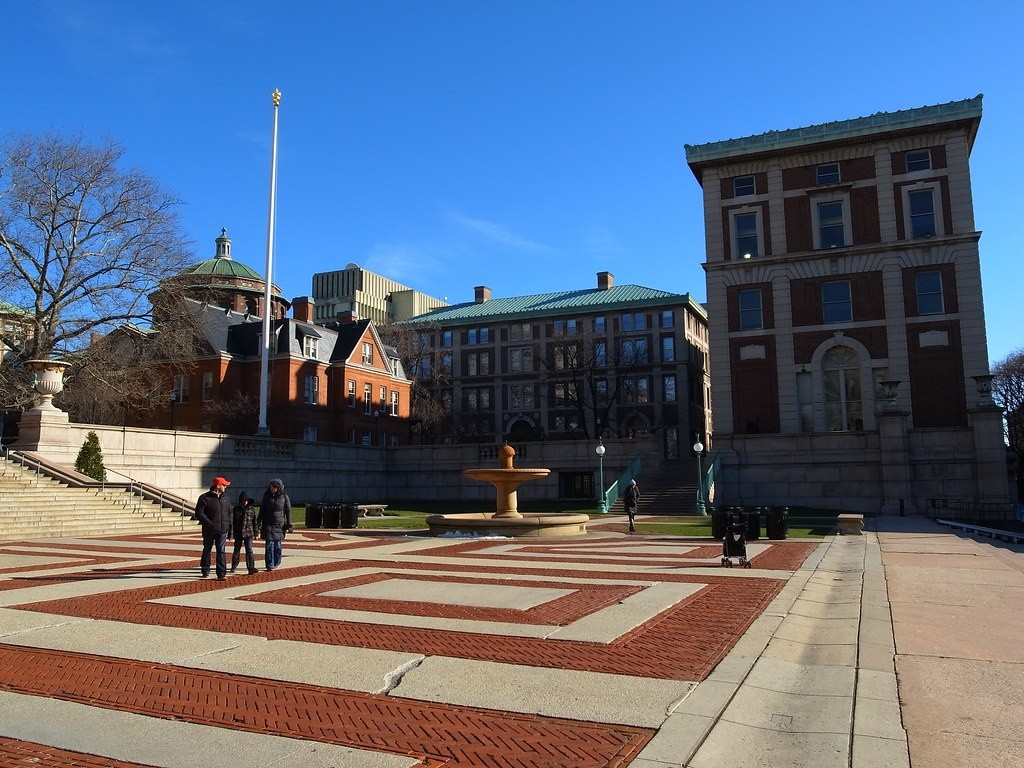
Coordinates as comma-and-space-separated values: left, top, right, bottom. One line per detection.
256, 476, 293, 572
226, 488, 260, 576
622, 479, 641, 532
194, 477, 234, 581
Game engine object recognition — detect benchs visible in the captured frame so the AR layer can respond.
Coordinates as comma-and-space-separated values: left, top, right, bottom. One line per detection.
837, 513, 865, 535
357, 504, 388, 518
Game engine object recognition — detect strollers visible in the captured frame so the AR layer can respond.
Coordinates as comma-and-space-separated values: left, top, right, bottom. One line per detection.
721, 514, 752, 569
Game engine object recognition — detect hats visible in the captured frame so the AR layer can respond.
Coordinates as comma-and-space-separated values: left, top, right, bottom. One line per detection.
212, 477, 231, 486
630, 479, 635, 485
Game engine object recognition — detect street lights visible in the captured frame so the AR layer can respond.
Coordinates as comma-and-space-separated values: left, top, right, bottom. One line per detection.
693, 434, 707, 516
596, 436, 608, 514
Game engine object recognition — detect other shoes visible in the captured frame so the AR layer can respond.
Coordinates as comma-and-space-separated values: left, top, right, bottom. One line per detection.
628, 526, 635, 531
273, 565, 278, 568
217, 575, 227, 580
267, 568, 272, 570
249, 568, 259, 575
201, 574, 210, 579
230, 567, 237, 573
